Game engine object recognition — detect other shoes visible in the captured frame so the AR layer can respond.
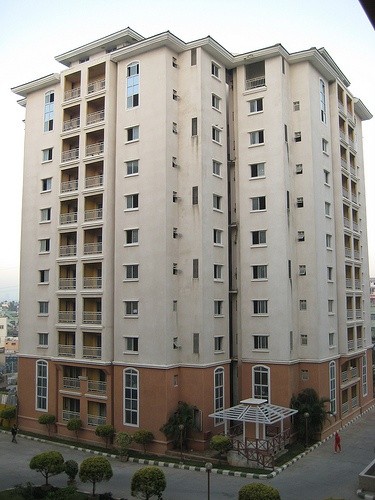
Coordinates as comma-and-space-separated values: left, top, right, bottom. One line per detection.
339, 450, 342, 453
334, 452, 337, 454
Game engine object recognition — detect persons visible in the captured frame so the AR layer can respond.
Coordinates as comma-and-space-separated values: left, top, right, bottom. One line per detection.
11, 423, 19, 444
333, 432, 342, 453
